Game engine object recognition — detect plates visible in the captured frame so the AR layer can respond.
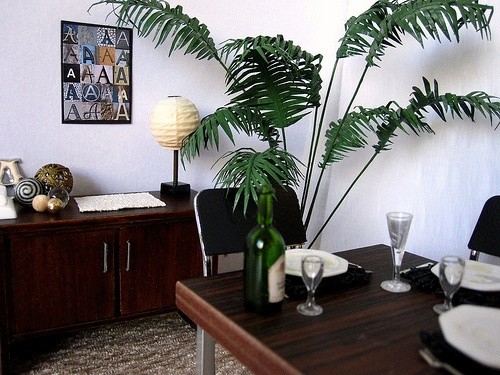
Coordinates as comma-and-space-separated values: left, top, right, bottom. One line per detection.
438, 303, 500, 369
285, 249, 349, 279
430, 260, 500, 291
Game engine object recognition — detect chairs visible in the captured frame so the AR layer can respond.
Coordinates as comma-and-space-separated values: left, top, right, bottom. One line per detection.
467, 194, 500, 264
193, 187, 306, 277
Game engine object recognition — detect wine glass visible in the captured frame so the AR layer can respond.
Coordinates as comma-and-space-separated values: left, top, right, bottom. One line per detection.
296, 254, 325, 317
433, 254, 467, 315
380, 211, 414, 293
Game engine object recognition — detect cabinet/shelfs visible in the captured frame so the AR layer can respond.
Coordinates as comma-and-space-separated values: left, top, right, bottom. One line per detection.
0, 190, 203, 375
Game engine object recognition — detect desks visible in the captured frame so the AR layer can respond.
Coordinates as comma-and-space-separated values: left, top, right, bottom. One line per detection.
175, 244, 454, 375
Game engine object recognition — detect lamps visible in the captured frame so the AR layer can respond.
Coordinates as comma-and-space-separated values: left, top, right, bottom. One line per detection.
150, 96, 200, 199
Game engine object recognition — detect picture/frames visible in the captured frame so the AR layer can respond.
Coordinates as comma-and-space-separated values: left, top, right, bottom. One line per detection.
60, 20, 134, 124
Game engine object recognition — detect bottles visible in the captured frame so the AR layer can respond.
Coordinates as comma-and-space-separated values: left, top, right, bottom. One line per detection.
243, 183, 285, 314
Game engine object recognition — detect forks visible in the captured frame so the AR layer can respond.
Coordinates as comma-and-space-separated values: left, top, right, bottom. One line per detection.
419, 348, 462, 375
